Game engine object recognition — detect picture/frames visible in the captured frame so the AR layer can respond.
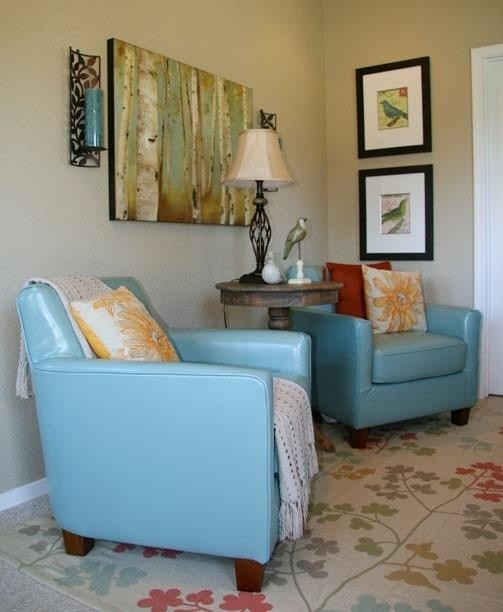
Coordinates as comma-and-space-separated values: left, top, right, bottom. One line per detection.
357, 165, 434, 262
356, 56, 432, 159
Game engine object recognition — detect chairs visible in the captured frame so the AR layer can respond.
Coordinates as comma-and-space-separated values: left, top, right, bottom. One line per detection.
287, 262, 481, 449
15, 275, 313, 589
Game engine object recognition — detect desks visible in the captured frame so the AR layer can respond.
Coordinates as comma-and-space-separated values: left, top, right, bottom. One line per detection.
216, 281, 344, 453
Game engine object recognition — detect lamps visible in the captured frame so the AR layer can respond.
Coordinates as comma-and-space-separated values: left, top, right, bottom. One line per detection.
220, 110, 294, 284
68, 47, 106, 167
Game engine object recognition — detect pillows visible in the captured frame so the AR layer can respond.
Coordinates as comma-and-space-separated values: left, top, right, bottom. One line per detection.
362, 264, 429, 335
321, 262, 392, 319
70, 285, 183, 367
